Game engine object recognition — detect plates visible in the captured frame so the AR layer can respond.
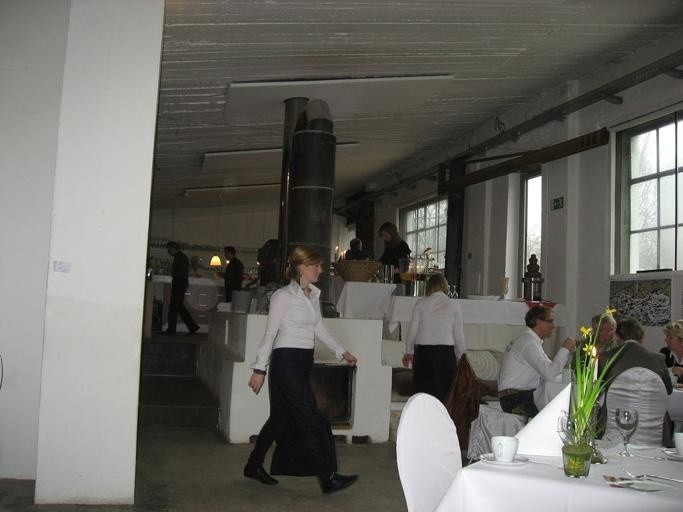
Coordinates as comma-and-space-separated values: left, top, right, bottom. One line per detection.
466, 293, 501, 301
477, 451, 531, 466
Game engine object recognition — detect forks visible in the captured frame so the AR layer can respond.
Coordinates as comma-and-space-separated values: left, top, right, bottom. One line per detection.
624, 468, 682, 483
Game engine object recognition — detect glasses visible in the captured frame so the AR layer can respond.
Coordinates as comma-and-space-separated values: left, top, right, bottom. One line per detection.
540, 318, 554, 323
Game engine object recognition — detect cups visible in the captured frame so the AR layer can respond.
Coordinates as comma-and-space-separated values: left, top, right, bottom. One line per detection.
490, 435, 519, 462
672, 431, 683, 456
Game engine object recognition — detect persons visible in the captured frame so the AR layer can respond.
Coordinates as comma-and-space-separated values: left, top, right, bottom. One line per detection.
400, 270, 467, 409
659, 319, 683, 384
493, 304, 577, 425
569, 314, 618, 380
216, 244, 247, 303
596, 318, 675, 443
377, 221, 412, 271
242, 243, 360, 495
160, 240, 201, 337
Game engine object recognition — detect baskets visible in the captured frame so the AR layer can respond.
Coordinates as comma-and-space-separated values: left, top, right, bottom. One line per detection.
333, 260, 381, 282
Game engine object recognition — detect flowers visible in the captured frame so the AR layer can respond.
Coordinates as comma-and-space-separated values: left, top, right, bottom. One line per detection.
558, 304, 631, 477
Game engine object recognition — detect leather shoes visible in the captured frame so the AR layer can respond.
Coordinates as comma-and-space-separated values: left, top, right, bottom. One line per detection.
320, 472, 357, 493
244, 463, 278, 485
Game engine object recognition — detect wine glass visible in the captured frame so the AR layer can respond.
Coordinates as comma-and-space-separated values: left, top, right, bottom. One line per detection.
614, 406, 638, 458
447, 285, 458, 299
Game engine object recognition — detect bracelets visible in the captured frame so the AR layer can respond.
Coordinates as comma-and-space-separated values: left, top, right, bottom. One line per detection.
253, 369, 267, 376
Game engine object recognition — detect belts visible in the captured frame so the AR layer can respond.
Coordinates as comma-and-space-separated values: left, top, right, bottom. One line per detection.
498, 389, 518, 399
